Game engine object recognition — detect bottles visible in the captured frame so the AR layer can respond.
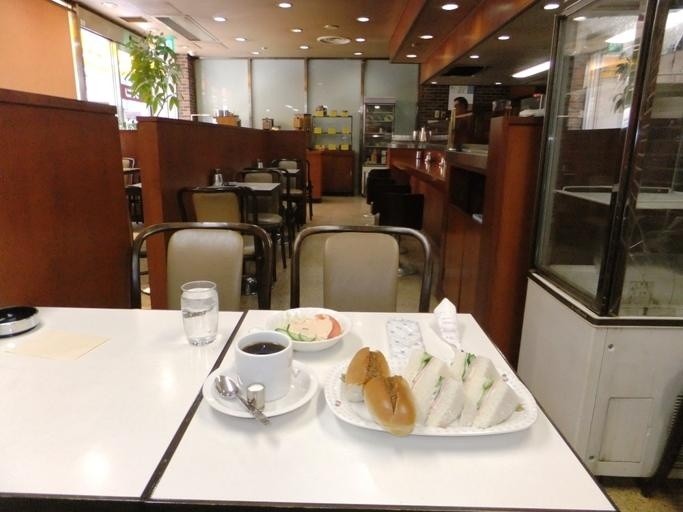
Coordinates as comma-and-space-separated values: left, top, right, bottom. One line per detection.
415, 148, 422, 159
213, 168, 223, 187
256, 159, 263, 170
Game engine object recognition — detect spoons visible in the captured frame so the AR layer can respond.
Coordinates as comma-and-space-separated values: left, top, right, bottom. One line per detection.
214, 372, 270, 427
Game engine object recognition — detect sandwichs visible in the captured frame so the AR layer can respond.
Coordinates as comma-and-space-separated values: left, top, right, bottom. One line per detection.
407, 350, 518, 429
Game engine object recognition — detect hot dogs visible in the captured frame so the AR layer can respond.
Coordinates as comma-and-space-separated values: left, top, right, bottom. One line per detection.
363, 375, 415, 436
343, 347, 390, 402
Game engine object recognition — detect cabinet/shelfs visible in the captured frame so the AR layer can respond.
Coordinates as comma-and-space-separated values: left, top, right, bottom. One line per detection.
361, 97, 397, 191
310, 113, 355, 195
510, 2, 683, 488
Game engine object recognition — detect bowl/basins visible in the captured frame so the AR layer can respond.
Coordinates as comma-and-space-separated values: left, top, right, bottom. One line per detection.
265, 307, 352, 357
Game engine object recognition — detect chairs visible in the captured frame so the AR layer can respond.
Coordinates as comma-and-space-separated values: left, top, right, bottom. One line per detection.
124, 157, 304, 309
366, 169, 425, 229
290, 225, 433, 311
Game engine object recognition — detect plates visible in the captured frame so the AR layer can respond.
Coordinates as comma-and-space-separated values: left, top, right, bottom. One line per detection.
330, 362, 539, 435
208, 361, 320, 420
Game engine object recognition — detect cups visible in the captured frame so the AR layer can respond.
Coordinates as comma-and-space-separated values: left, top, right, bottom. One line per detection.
179, 279, 220, 347
236, 328, 293, 400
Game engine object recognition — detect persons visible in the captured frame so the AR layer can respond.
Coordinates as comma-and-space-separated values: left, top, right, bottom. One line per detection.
454, 97, 469, 116
